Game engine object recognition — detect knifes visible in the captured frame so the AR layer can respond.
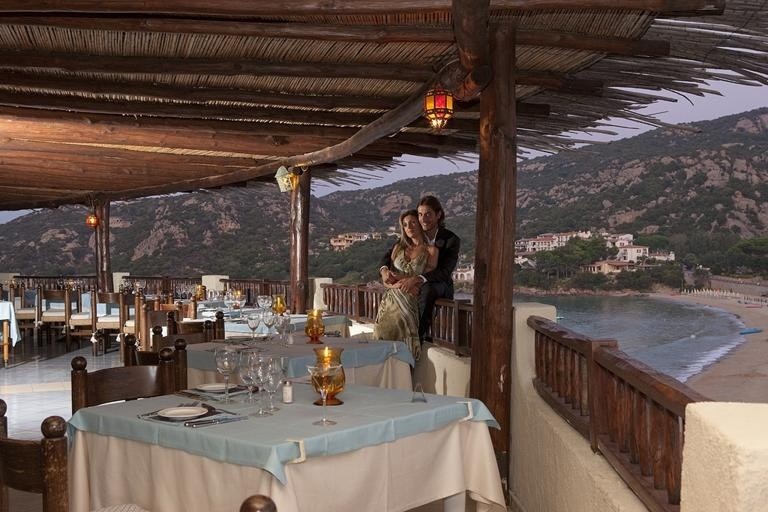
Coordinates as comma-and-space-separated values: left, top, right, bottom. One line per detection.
183, 416, 247, 427
192, 419, 247, 427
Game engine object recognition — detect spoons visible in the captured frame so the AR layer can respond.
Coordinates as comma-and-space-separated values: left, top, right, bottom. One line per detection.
201, 403, 237, 416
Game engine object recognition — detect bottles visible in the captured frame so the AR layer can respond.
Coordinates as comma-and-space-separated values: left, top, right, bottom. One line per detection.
0, 280, 26, 290
287, 329, 294, 345
282, 380, 294, 403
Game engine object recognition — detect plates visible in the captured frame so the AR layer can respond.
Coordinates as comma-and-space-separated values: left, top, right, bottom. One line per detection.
226, 336, 251, 342
157, 405, 208, 421
196, 382, 241, 393
224, 344, 249, 349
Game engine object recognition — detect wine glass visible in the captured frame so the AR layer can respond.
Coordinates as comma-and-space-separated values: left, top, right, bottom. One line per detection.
248, 313, 261, 345
119, 278, 149, 298
273, 315, 292, 350
305, 362, 344, 427
304, 309, 325, 344
216, 348, 238, 405
310, 347, 347, 406
238, 349, 291, 420
262, 311, 279, 346
175, 279, 288, 324
56, 273, 89, 293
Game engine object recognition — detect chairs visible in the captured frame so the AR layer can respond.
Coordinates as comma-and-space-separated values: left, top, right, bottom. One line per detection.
70, 348, 176, 415
1, 400, 67, 512
2, 277, 225, 365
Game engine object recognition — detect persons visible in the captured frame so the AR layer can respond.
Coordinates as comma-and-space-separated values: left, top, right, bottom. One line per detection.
373, 210, 439, 361
380, 194, 460, 343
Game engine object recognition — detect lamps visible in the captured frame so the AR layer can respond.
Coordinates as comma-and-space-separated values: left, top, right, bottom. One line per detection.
86, 213, 98, 229
423, 76, 455, 132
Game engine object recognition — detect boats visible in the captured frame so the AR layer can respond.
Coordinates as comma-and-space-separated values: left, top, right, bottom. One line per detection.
740, 328, 761, 335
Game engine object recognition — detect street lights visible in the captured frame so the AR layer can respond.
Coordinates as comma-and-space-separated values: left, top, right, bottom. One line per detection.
737, 278, 744, 296
556, 315, 565, 321
757, 282, 762, 298
